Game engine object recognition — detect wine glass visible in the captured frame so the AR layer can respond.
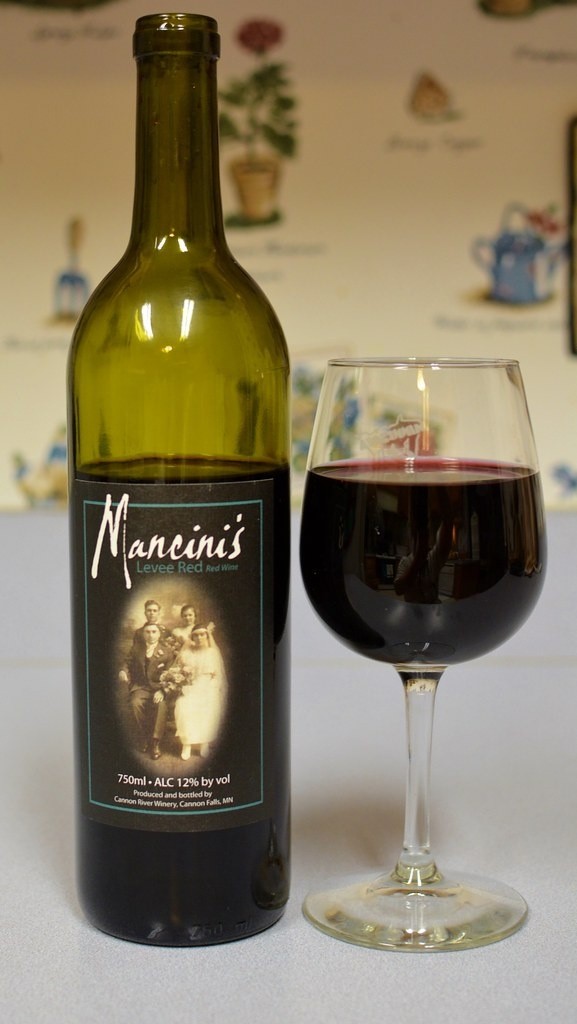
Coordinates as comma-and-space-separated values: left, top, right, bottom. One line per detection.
298, 358, 548, 954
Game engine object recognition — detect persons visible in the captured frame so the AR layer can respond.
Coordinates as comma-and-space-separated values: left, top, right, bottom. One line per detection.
117, 599, 227, 761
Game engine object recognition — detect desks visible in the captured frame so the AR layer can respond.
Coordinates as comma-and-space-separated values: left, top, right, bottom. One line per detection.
0, 508, 577, 1024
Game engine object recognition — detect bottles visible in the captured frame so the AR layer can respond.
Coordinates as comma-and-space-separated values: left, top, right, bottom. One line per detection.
67, 12, 291, 949
